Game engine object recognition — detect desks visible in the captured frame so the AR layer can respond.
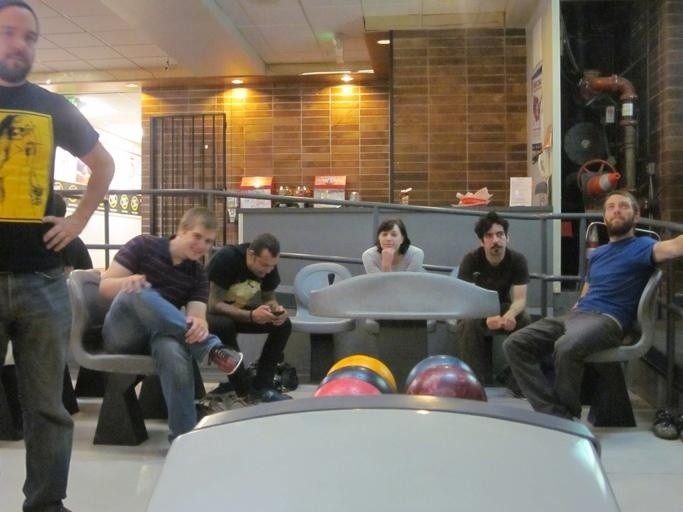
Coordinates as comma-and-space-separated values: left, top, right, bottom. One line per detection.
304, 271, 496, 387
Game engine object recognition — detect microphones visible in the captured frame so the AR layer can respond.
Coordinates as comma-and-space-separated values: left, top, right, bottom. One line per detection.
327, 273, 335, 285
472, 271, 481, 285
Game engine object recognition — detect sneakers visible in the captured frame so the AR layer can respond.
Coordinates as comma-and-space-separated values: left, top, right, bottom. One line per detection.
674, 414, 683, 439
652, 408, 678, 439
210, 345, 244, 376
258, 384, 292, 401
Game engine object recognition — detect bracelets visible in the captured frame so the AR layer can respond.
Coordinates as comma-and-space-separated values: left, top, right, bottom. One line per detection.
249, 309, 254, 324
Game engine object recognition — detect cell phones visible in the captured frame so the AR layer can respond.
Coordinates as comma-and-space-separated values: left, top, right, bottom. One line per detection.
271, 310, 287, 315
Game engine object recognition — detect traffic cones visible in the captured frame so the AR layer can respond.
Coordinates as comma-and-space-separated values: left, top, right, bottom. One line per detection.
580, 168, 621, 206
584, 225, 599, 261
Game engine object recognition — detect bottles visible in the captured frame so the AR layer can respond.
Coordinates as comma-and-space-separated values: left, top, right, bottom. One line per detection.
278, 185, 309, 208
400, 188, 408, 204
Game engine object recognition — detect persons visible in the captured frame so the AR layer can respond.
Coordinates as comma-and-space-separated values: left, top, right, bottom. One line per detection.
43, 193, 93, 270
360, 215, 429, 394
457, 207, 534, 401
205, 232, 293, 406
98, 205, 245, 445
501, 188, 683, 422
1, 1, 116, 512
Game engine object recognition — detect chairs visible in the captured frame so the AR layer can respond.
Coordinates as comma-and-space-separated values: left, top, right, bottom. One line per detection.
146, 396, 617, 511
283, 262, 354, 383
67, 266, 158, 445
445, 264, 461, 333
578, 265, 664, 427
363, 317, 437, 335
